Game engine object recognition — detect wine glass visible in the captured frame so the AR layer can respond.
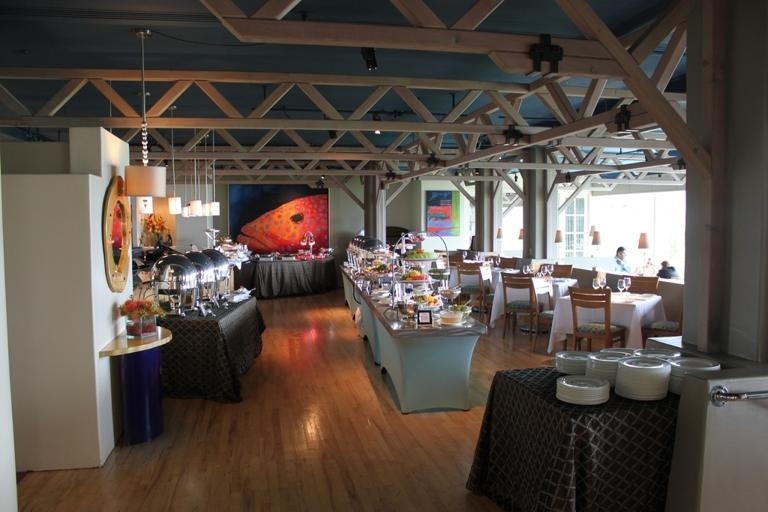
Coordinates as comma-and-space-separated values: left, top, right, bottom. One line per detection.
591, 276, 631, 298
462, 249, 500, 269
521, 264, 555, 281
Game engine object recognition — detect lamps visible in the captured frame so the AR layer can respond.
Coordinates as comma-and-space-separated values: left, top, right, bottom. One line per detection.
497, 225, 651, 248
300, 231, 315, 254
125, 27, 220, 218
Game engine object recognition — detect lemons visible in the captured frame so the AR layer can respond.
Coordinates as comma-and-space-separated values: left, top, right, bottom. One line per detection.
428, 295, 433, 302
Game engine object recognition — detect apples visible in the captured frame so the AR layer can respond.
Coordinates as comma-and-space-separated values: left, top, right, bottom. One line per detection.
428, 302, 439, 307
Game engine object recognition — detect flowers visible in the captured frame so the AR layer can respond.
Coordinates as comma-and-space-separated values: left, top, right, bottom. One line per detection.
141, 213, 168, 248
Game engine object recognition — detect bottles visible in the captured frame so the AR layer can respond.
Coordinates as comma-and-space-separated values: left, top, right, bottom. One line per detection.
318, 247, 326, 258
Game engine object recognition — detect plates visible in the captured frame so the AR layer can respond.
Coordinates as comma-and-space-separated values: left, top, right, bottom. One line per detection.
435, 320, 468, 326
553, 346, 720, 406
372, 289, 434, 305
422, 303, 443, 309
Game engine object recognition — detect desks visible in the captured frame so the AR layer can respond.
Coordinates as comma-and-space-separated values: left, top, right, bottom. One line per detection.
99, 326, 174, 447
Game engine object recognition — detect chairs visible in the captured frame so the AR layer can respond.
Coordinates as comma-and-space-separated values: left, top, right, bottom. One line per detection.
434, 249, 685, 355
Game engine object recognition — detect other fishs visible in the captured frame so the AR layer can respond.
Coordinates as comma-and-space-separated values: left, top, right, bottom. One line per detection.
236, 193, 328, 256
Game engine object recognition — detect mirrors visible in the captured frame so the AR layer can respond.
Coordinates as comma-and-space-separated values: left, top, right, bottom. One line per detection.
102, 176, 132, 293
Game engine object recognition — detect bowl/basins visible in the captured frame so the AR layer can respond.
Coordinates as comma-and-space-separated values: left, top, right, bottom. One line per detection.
439, 311, 462, 322
448, 304, 473, 317
397, 303, 417, 316
436, 286, 459, 300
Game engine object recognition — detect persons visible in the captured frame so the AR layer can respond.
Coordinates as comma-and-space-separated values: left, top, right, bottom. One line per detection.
614, 247, 631, 275
657, 261, 677, 278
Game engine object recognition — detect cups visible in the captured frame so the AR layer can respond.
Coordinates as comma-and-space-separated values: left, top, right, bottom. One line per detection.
361, 280, 372, 294
297, 252, 311, 260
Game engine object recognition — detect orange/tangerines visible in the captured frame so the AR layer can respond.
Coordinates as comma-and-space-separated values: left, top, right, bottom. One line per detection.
409, 270, 419, 276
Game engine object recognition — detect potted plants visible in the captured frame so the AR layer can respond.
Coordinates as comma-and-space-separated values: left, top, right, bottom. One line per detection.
121, 298, 166, 341
